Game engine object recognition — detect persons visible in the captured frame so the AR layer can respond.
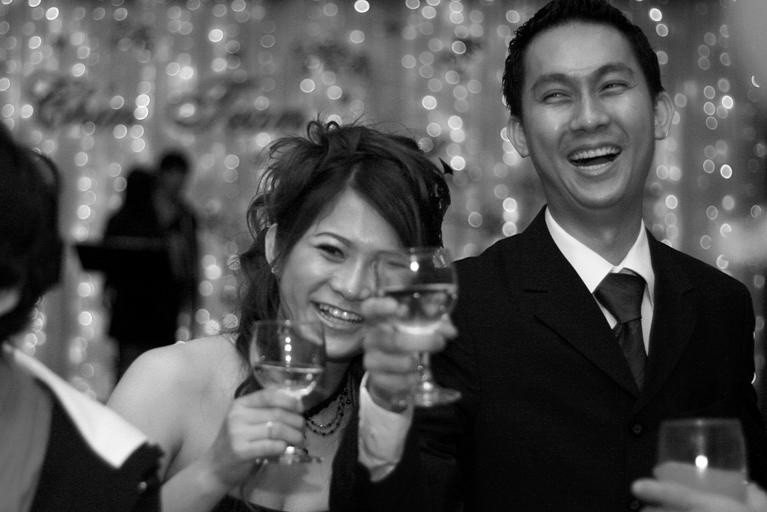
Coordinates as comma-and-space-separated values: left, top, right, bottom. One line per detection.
101, 166, 183, 388
631, 455, 767, 510
325, 1, 767, 510
104, 117, 452, 512
0, 119, 165, 510
152, 146, 202, 337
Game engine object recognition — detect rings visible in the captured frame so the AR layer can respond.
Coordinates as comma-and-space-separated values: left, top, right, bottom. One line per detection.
265, 419, 273, 438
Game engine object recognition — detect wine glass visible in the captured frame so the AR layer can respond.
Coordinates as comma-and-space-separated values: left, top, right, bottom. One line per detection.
657, 415, 750, 505
248, 318, 324, 468
374, 243, 464, 411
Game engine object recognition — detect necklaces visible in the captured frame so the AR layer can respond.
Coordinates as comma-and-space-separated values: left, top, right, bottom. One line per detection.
302, 367, 355, 436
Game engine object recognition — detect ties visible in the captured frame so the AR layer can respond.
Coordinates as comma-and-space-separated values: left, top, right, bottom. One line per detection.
595, 273, 648, 392
